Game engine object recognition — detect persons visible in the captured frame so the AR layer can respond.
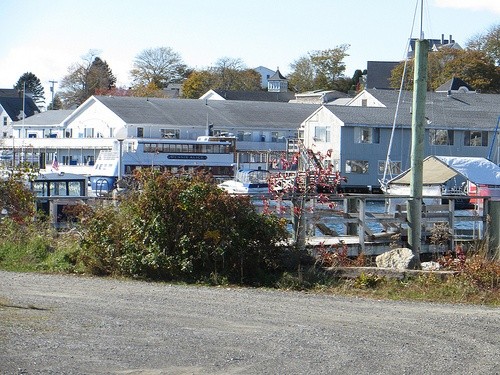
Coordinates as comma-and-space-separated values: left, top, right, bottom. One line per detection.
88, 157, 94, 166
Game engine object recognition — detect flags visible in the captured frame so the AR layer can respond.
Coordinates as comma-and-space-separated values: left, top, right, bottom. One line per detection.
52, 152, 59, 171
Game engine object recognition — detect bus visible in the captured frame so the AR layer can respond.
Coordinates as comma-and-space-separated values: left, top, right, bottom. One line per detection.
89, 136, 239, 201
